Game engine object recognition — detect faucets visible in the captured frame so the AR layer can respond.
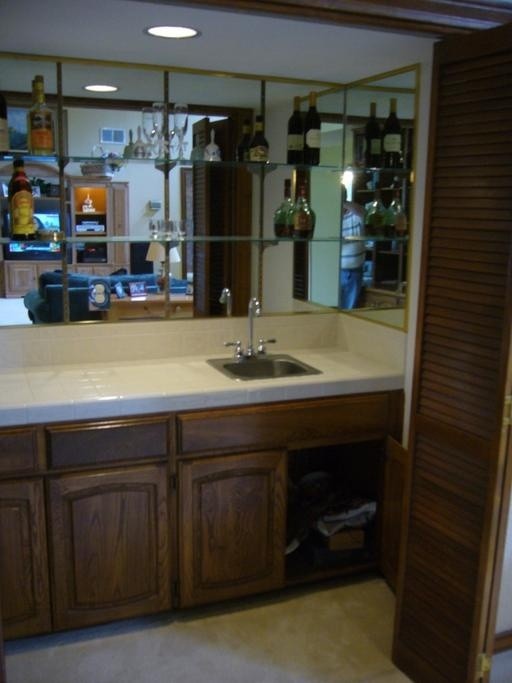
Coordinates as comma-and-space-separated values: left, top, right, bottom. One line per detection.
218, 287, 234, 316
244, 295, 263, 357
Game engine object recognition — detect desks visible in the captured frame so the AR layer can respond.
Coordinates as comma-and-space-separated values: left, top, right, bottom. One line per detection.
101, 293, 194, 321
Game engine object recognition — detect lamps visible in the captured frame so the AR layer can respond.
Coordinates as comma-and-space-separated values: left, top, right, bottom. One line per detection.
145, 242, 182, 292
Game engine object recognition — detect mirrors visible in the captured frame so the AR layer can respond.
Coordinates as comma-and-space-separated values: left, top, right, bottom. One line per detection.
1, 52, 420, 328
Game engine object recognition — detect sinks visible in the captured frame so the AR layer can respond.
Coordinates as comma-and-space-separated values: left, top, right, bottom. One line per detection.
204, 349, 323, 383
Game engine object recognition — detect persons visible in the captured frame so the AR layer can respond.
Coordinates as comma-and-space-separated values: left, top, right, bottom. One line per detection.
341, 185, 368, 309
23, 216, 49, 248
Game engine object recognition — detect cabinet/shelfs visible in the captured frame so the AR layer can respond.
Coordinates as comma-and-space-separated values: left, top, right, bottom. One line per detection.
2, 162, 129, 299
0, 51, 347, 324
340, 122, 415, 308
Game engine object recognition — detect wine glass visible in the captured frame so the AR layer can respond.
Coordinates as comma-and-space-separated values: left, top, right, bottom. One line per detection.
142, 107, 153, 158
162, 217, 186, 240
152, 104, 189, 161
148, 218, 161, 238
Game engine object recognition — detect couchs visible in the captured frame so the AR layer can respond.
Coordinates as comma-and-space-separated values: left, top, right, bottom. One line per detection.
24, 284, 188, 324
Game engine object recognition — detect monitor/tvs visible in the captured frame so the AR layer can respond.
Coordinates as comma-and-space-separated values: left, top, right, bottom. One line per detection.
4, 211, 63, 260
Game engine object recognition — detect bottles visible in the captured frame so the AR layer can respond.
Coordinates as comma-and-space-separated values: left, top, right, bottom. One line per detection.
363, 102, 381, 166
272, 179, 294, 238
27, 80, 35, 152
288, 179, 318, 240
363, 183, 386, 235
383, 98, 403, 166
286, 95, 301, 164
1, 94, 10, 152
302, 92, 322, 165
251, 116, 269, 161
8, 157, 37, 241
30, 76, 57, 154
237, 116, 250, 162
385, 182, 408, 238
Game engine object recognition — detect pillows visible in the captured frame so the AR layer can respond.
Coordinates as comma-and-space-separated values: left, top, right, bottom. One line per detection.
39, 268, 188, 297
